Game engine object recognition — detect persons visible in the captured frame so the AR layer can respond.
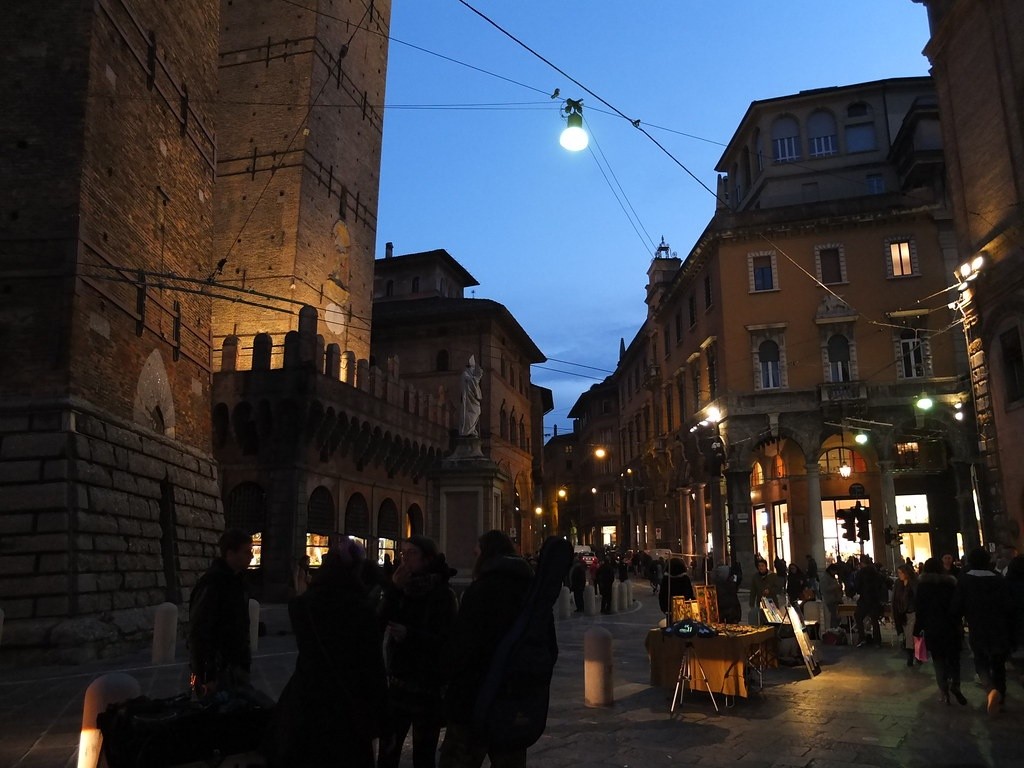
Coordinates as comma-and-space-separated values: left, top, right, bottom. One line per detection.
186, 529, 1024, 768
458, 352, 483, 438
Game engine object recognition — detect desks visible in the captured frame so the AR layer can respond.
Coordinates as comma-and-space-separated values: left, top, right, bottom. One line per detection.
97, 685, 286, 768
835, 604, 896, 649
773, 622, 820, 665
647, 624, 776, 705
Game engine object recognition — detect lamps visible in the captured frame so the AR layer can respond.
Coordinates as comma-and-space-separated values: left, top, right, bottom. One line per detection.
840, 461, 851, 479
916, 392, 933, 409
855, 429, 868, 445
558, 97, 588, 151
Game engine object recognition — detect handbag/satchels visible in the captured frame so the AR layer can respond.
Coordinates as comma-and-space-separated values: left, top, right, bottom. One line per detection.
912, 629, 928, 663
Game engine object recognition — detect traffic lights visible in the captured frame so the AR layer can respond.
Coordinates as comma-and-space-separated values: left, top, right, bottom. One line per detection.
883, 525, 904, 549
853, 506, 870, 541
836, 507, 857, 542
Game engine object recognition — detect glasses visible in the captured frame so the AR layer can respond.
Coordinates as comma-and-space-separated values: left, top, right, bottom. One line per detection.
397, 550, 422, 560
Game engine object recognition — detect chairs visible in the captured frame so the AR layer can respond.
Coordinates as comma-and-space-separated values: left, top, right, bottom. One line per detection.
747, 608, 773, 671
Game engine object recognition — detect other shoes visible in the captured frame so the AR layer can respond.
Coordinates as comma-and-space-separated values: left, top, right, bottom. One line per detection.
986, 689, 1001, 714
856, 639, 866, 647
906, 655, 913, 667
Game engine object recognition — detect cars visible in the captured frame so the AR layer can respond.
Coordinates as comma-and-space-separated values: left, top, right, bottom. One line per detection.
573, 543, 671, 577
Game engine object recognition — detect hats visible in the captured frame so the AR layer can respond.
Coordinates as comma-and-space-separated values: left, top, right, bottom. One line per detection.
406, 535, 438, 558
